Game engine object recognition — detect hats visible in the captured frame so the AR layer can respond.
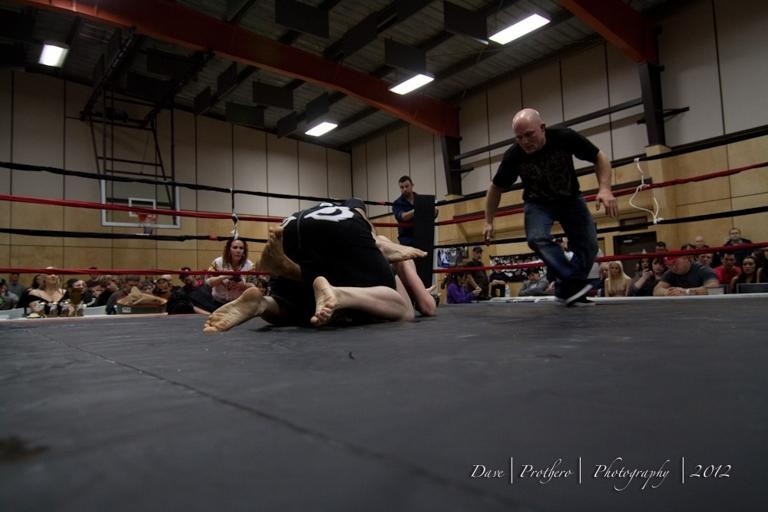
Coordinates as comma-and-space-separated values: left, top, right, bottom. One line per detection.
663, 249, 684, 267
473, 247, 482, 252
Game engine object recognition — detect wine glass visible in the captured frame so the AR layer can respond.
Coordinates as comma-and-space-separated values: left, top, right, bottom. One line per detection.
68, 288, 83, 317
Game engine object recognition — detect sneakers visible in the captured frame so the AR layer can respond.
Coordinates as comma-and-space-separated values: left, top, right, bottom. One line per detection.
562, 283, 596, 307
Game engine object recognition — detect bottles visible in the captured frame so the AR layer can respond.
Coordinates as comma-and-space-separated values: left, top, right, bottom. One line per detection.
505, 284, 512, 297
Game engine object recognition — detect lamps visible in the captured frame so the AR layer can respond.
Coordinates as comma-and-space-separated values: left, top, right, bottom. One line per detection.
485, 1, 550, 44
37, 38, 68, 70
305, 116, 339, 138
387, 60, 435, 96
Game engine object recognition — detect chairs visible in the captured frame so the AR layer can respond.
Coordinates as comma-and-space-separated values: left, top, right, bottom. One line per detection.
0, 304, 111, 317
